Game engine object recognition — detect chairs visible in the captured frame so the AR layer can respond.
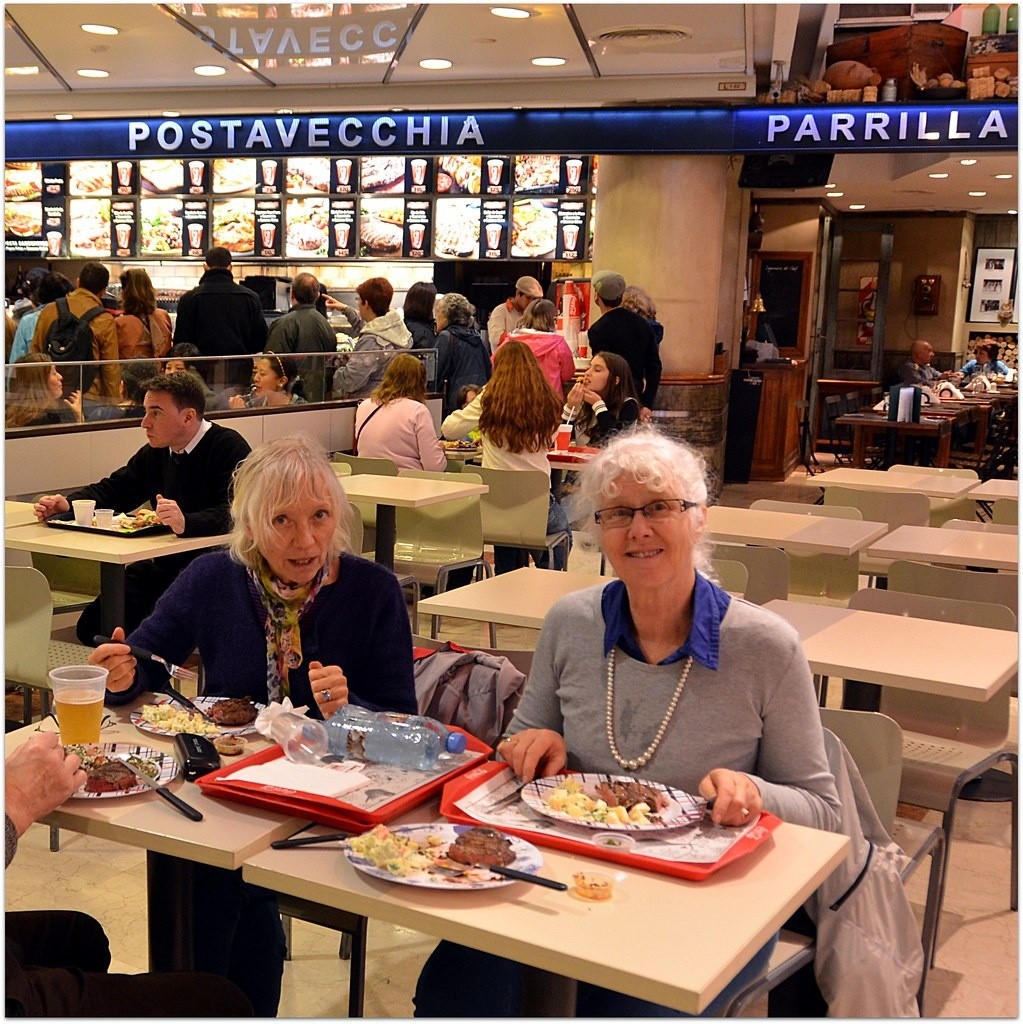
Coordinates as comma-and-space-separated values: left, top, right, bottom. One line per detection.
890, 557, 1017, 704
704, 558, 749, 595
461, 464, 570, 577
703, 539, 790, 606
814, 703, 947, 1020
847, 587, 1020, 1015
820, 483, 933, 587
941, 518, 1019, 539
751, 497, 866, 611
25, 491, 102, 615
823, 391, 884, 468
699, 724, 848, 1017
5, 565, 98, 726
887, 464, 980, 575
331, 450, 398, 530
392, 469, 498, 650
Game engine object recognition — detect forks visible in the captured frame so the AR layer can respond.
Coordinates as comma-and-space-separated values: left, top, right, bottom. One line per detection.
238, 386, 258, 398
431, 862, 568, 892
94, 635, 200, 684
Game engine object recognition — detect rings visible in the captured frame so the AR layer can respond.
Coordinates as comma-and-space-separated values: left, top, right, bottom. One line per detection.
741, 806, 750, 816
504, 736, 518, 745
321, 690, 332, 702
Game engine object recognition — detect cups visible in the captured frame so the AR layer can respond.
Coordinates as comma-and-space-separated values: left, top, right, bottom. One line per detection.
94, 509, 114, 528
269, 713, 329, 766
41, 149, 584, 259
555, 424, 573, 455
72, 500, 96, 526
556, 279, 589, 359
49, 664, 110, 745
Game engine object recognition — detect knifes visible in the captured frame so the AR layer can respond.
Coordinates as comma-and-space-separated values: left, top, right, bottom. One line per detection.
270, 825, 438, 850
164, 687, 214, 723
118, 757, 203, 821
485, 770, 542, 807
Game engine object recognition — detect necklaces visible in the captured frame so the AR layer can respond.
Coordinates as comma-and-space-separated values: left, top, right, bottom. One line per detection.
607, 643, 695, 771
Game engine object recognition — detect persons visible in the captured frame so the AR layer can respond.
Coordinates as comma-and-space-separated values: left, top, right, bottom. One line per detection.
455, 384, 484, 412
87, 430, 420, 1017
891, 339, 953, 389
620, 285, 663, 355
584, 270, 663, 429
403, 281, 436, 357
319, 276, 415, 403
487, 276, 545, 370
983, 279, 1002, 294
356, 354, 449, 474
408, 423, 844, 1018
985, 258, 1004, 269
4, 343, 314, 434
114, 269, 174, 381
170, 246, 270, 390
264, 273, 339, 401
31, 263, 124, 402
487, 297, 575, 415
425, 291, 495, 423
553, 350, 643, 529
5, 731, 112, 1018
30, 370, 254, 650
948, 338, 1014, 388
980, 298, 1000, 313
439, 341, 574, 576
5, 268, 75, 363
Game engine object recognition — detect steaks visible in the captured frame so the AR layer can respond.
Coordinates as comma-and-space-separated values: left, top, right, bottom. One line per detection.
448, 829, 516, 866
84, 762, 137, 793
206, 698, 259, 726
592, 779, 670, 812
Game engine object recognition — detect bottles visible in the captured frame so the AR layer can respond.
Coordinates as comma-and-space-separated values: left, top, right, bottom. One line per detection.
301, 715, 440, 771
333, 704, 468, 755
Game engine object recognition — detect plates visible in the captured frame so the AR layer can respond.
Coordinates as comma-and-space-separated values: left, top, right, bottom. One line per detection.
521, 772, 706, 831
344, 824, 545, 891
445, 444, 481, 451
60, 742, 181, 799
130, 695, 268, 739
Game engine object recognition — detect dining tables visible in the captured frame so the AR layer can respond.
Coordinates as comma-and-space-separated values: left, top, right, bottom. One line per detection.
760, 599, 1019, 703
417, 564, 618, 633
5, 498, 43, 527
808, 467, 981, 501
701, 505, 889, 558
967, 477, 1019, 501
550, 455, 606, 577
5, 683, 317, 879
337, 471, 491, 570
241, 791, 852, 1019
835, 371, 1018, 481
871, 523, 1018, 572
5, 517, 237, 646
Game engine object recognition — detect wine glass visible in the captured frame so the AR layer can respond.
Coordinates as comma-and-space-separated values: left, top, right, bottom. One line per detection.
971, 373, 980, 395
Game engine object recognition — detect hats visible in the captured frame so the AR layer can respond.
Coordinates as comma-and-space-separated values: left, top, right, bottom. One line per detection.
515, 276, 544, 297
591, 269, 626, 300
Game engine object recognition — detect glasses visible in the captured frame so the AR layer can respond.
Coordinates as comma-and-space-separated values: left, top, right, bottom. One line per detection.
356, 297, 365, 302
593, 500, 701, 529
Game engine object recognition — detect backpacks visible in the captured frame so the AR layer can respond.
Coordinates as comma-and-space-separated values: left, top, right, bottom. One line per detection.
42, 296, 108, 398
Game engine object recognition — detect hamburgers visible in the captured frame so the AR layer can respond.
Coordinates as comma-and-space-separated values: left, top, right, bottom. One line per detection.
5, 155, 598, 260
